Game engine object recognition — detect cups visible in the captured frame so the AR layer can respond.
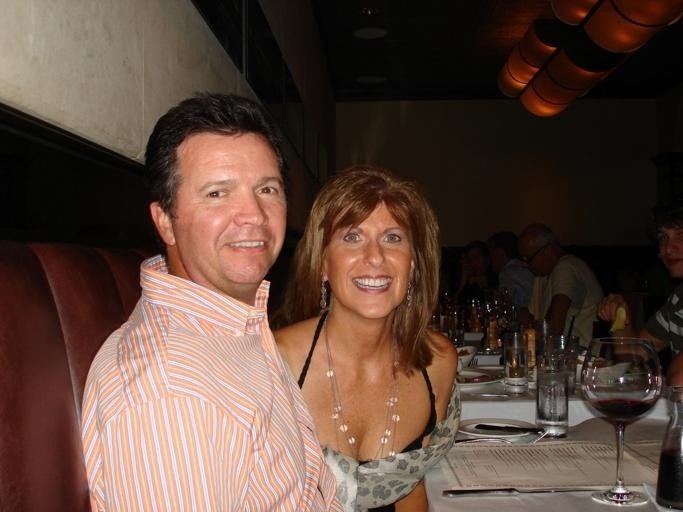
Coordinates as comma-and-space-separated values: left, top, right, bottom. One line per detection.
504, 332, 529, 398
548, 334, 577, 395
447, 310, 464, 348
535, 353, 569, 440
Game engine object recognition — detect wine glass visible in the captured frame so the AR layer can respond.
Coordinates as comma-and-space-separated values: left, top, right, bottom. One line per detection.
580, 336, 663, 505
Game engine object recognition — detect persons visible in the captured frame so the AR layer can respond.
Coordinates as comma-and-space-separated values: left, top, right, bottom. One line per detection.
596, 200, 683, 401
80, 91, 344, 512
461, 223, 604, 349
271, 167, 462, 512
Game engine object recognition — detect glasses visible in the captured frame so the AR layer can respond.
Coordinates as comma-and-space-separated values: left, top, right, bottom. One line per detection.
522, 242, 549, 266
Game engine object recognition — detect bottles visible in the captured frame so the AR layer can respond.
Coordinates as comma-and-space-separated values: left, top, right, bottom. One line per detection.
654, 383, 683, 511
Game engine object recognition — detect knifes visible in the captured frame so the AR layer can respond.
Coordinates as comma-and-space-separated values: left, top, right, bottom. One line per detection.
442, 487, 603, 498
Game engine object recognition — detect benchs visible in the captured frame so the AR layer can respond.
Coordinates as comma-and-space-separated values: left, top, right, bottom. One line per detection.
0, 239, 152, 512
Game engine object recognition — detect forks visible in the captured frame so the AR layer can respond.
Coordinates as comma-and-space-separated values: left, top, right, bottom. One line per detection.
468, 358, 478, 369
454, 430, 548, 446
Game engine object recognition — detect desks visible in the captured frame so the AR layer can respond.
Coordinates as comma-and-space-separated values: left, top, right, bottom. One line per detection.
394, 340, 663, 512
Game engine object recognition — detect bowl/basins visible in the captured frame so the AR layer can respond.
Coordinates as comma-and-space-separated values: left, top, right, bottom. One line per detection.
456, 345, 477, 369
576, 355, 632, 387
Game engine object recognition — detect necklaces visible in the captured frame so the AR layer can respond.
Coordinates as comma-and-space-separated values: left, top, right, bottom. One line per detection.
323, 314, 402, 460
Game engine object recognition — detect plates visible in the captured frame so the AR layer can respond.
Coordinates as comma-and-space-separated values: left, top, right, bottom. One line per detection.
456, 367, 505, 388
457, 418, 536, 443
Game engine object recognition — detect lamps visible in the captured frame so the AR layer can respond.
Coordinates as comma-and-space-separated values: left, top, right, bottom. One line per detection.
497, 0, 683, 119
